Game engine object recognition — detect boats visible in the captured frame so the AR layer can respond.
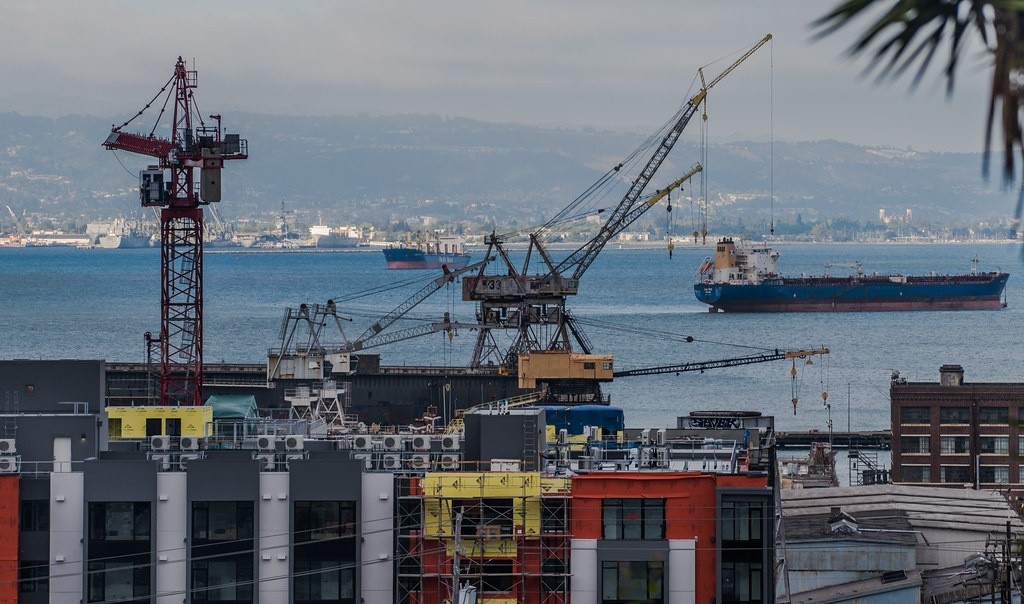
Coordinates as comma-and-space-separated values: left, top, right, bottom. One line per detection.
382, 230, 472, 270
689, 234, 1010, 311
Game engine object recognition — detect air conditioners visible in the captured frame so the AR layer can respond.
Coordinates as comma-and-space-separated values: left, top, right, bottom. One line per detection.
590, 426, 598, 459
412, 455, 430, 468
412, 435, 431, 449
180, 455, 198, 469
641, 428, 666, 466
442, 455, 459, 468
257, 455, 275, 468
286, 435, 304, 449
286, 455, 303, 468
151, 435, 170, 449
384, 454, 401, 468
442, 435, 460, 449
354, 454, 372, 468
180, 436, 199, 449
152, 455, 170, 468
0, 455, 20, 471
257, 435, 275, 449
354, 435, 371, 449
0, 438, 16, 453
384, 435, 401, 449
560, 429, 567, 465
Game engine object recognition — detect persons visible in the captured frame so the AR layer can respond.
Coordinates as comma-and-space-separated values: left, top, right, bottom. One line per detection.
758, 271, 784, 284
684, 465, 688, 470
708, 276, 712, 280
375, 424, 380, 435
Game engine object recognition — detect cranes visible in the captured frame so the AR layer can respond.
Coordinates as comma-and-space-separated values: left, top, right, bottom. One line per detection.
101, 55, 249, 408
268, 32, 773, 370
